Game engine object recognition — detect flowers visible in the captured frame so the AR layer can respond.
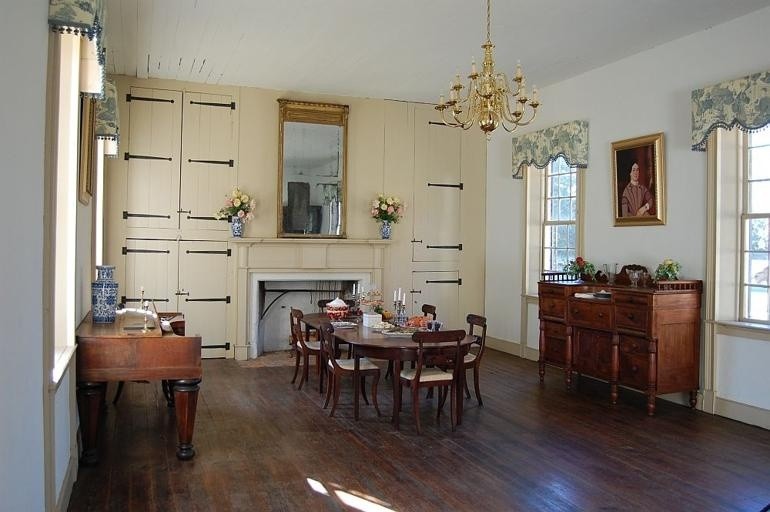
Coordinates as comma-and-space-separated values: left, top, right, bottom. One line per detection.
654, 258, 682, 280
370, 191, 407, 224
563, 256, 596, 278
360, 290, 385, 306
214, 186, 257, 223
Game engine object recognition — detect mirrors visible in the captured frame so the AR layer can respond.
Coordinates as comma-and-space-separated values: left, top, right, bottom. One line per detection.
275, 97, 351, 239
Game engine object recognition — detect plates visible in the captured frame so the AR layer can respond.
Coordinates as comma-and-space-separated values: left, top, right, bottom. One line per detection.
331, 321, 357, 330
382, 329, 416, 337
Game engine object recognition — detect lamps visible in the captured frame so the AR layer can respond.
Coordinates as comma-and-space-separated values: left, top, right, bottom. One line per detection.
434, 0, 542, 142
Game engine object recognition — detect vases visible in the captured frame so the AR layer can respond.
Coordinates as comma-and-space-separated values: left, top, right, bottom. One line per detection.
230, 217, 245, 239
91, 265, 119, 324
379, 220, 393, 240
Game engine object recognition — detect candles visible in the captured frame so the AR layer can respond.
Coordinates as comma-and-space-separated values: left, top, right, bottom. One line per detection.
352, 284, 355, 295
403, 293, 405, 305
394, 291, 396, 301
398, 288, 401, 300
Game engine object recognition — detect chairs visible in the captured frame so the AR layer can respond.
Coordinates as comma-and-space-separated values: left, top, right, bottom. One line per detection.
290, 299, 488, 434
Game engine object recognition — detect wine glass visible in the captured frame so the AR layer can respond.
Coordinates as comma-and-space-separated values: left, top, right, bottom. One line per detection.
629, 271, 652, 288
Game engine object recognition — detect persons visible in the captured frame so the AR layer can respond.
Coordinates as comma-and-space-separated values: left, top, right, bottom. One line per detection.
621, 159, 653, 216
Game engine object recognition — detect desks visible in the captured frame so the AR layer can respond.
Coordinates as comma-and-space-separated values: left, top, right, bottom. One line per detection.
74, 310, 203, 461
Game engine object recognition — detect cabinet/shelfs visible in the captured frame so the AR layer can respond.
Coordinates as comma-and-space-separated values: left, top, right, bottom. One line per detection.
410, 104, 464, 331
121, 85, 236, 359
537, 271, 703, 417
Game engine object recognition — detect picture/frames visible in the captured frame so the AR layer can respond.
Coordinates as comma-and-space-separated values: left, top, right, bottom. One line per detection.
79, 96, 96, 207
611, 130, 670, 227
